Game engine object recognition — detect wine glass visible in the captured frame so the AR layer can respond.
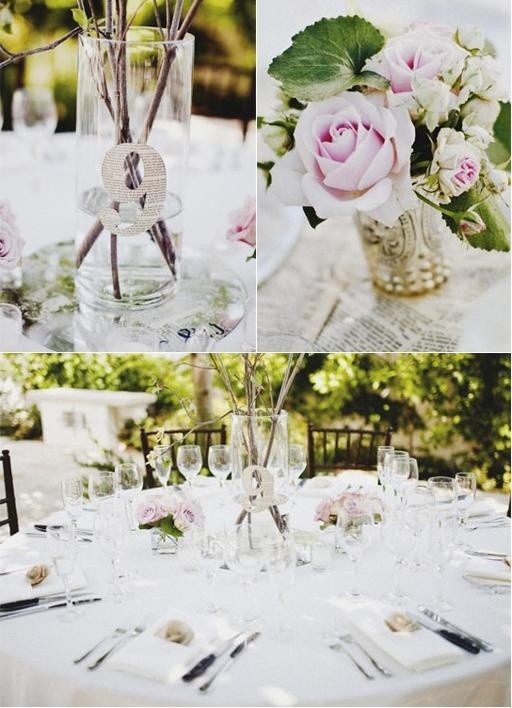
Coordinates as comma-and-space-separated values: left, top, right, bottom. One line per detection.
42, 446, 511, 607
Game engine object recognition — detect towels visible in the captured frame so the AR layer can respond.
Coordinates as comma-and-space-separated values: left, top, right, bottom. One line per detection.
458, 558, 511, 587
105, 630, 198, 682
369, 628, 465, 673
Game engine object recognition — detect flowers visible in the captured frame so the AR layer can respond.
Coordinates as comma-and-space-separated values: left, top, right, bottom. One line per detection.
137, 498, 199, 537
257, 15, 511, 251
314, 486, 382, 530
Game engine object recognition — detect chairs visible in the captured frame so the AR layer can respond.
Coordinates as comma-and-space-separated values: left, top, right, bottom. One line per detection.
308, 423, 393, 479
0, 450, 19, 536
140, 424, 226, 489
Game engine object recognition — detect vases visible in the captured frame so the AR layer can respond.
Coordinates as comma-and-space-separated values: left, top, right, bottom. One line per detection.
230, 408, 288, 556
150, 530, 177, 554
74, 25, 195, 313
354, 173, 448, 297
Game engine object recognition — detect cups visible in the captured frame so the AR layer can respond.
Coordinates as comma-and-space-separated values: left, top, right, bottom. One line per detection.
0, 301, 24, 354
10, 86, 60, 146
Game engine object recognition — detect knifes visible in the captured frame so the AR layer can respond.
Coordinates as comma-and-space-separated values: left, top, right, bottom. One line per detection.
406, 611, 479, 653
320, 625, 391, 679
182, 630, 261, 690
419, 604, 492, 652
73, 620, 149, 669
1, 586, 104, 621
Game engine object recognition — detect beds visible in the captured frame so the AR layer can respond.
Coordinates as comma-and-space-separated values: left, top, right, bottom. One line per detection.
0, 477, 511, 707
